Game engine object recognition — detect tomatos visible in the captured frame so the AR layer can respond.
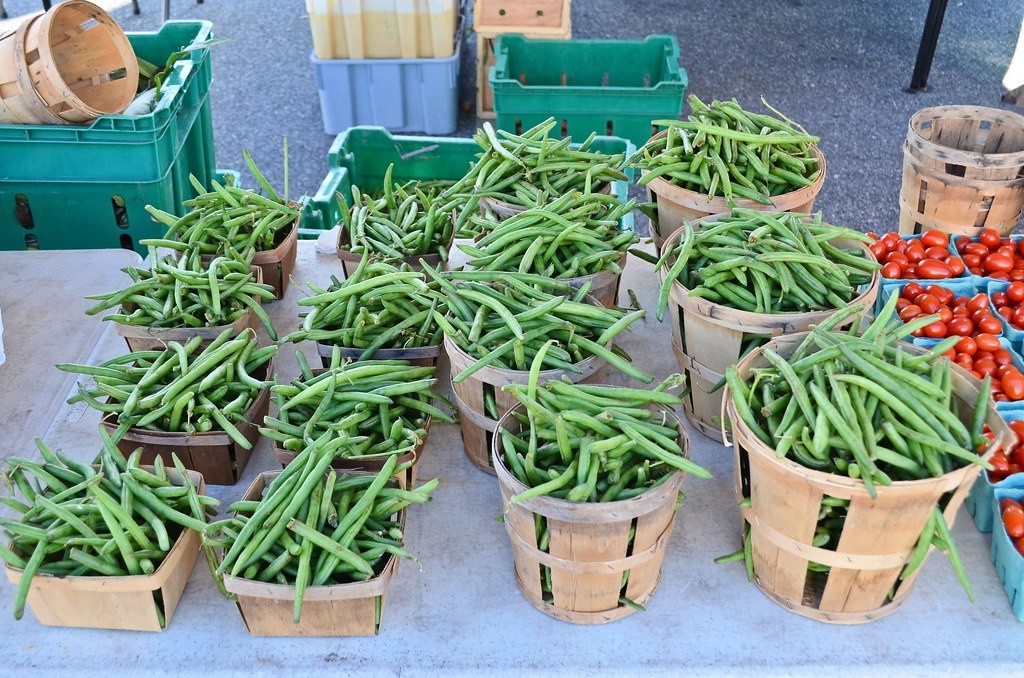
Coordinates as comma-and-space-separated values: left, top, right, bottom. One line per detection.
862, 229, 1024, 557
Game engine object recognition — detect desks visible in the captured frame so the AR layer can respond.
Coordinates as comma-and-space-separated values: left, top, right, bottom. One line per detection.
0, 231, 1024, 678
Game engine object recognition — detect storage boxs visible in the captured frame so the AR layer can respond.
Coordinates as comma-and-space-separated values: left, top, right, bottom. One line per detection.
872, 234, 1024, 625
310, 17, 466, 137
274, 364, 430, 487
180, 208, 299, 305
216, 467, 403, 637
96, 350, 272, 479
306, 0, 458, 58
487, 31, 688, 152
5, 461, 206, 631
113, 265, 264, 353
300, 118, 636, 243
473, 0, 573, 121
339, 222, 455, 280
303, 279, 439, 373
0, 17, 242, 256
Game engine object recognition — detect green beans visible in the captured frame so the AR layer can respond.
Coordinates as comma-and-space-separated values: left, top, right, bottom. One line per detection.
0, 96, 990, 631
